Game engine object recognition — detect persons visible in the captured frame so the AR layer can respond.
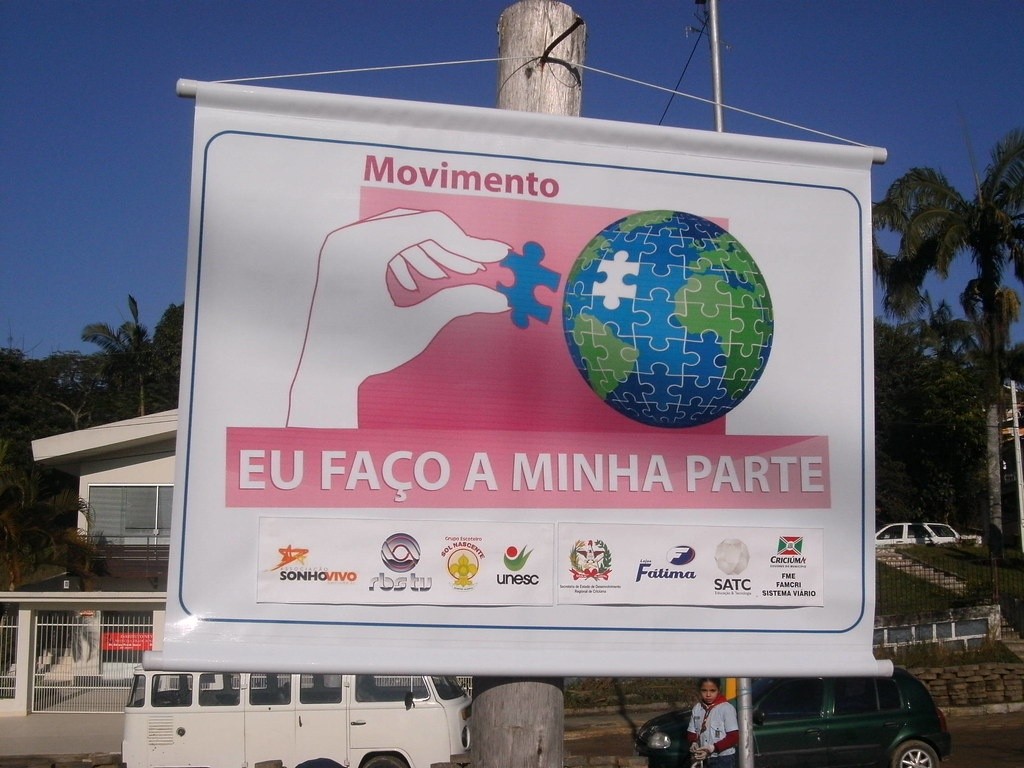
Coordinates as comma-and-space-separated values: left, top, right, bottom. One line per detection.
686, 676, 740, 767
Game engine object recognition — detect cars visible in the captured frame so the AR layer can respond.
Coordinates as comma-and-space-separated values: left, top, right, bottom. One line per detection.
873, 521, 984, 549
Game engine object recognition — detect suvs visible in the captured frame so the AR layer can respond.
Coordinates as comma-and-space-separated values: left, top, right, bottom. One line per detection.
635, 668, 952, 768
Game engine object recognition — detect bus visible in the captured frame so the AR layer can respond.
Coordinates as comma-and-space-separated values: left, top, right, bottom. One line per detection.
119, 660, 477, 768
